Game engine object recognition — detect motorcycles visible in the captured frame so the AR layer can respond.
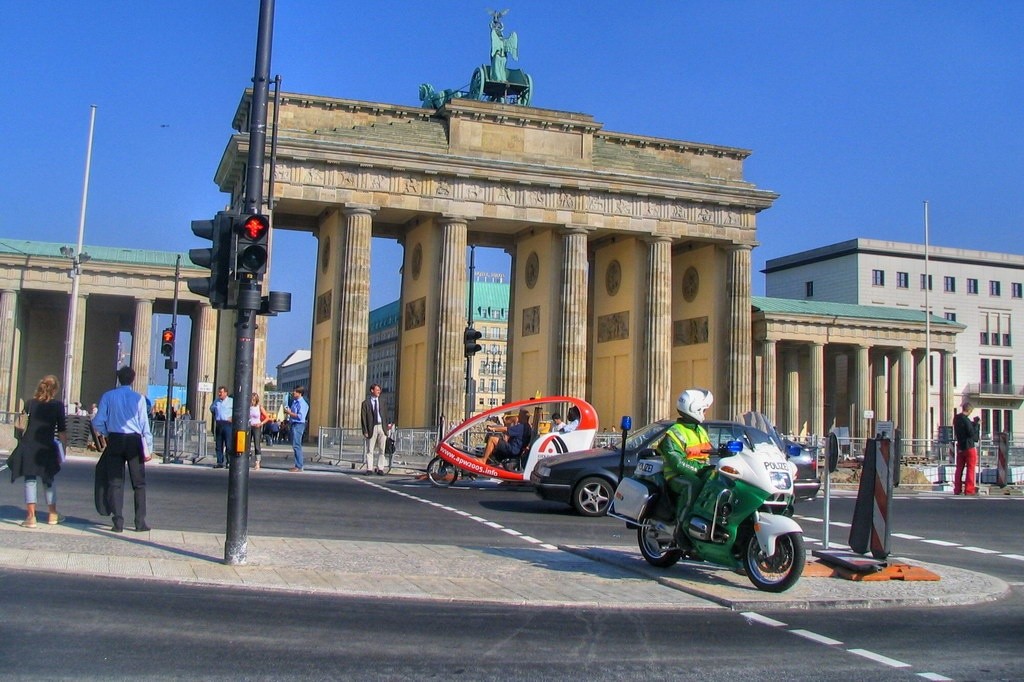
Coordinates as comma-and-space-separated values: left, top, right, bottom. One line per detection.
605, 411, 807, 593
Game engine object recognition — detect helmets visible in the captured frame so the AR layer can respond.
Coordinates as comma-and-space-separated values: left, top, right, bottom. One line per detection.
676, 388, 713, 424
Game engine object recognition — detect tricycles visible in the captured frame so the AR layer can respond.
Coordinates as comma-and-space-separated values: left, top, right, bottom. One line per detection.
425, 389, 600, 490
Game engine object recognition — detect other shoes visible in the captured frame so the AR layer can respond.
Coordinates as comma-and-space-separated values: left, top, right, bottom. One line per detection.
377, 470, 385, 476
474, 457, 487, 465
111, 525, 122, 532
213, 463, 223, 469
673, 528, 700, 555
254, 462, 260, 469
21, 516, 36, 528
136, 525, 151, 532
48, 513, 65, 524
289, 466, 302, 472
363, 470, 372, 476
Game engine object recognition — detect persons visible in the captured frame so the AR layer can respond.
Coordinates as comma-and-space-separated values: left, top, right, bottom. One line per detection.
388, 420, 395, 437
210, 386, 233, 468
548, 406, 581, 433
780, 430, 812, 445
657, 390, 716, 554
448, 421, 457, 431
261, 419, 304, 444
74, 402, 192, 438
285, 384, 309, 473
6, 374, 67, 529
489, 21, 507, 82
953, 402, 980, 496
91, 365, 153, 533
249, 393, 270, 469
361, 383, 389, 476
600, 426, 618, 449
474, 409, 532, 465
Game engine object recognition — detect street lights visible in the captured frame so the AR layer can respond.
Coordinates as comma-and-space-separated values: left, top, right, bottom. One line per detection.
58, 102, 101, 414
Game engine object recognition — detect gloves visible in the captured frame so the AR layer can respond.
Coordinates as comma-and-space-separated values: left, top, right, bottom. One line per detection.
694, 465, 716, 479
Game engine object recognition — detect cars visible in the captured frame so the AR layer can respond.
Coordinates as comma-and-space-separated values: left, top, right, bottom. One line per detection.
530, 418, 823, 518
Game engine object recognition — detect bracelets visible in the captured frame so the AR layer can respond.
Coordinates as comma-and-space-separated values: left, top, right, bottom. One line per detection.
261, 422, 264, 425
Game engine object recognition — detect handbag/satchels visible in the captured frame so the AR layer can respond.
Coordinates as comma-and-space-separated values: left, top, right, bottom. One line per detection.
14, 398, 32, 439
384, 437, 396, 454
260, 405, 265, 422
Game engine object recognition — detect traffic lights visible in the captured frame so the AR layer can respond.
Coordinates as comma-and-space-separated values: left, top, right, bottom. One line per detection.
235, 212, 270, 281
256, 290, 291, 316
185, 210, 239, 311
463, 327, 483, 357
161, 327, 176, 358
